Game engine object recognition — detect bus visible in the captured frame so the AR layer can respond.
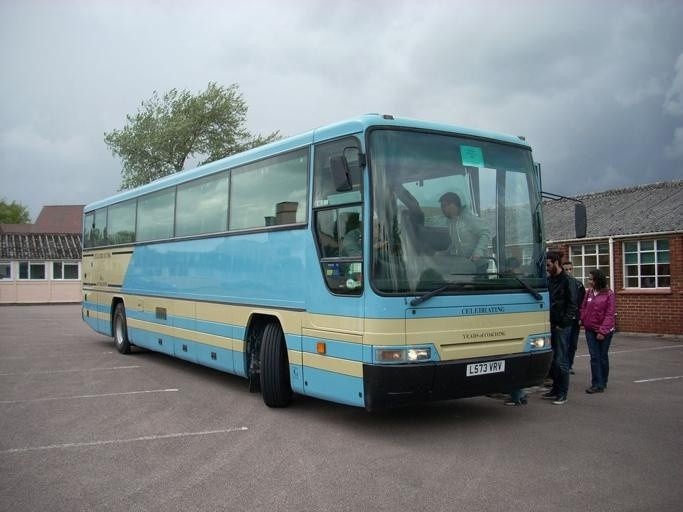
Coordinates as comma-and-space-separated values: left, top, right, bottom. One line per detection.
82, 113, 586, 411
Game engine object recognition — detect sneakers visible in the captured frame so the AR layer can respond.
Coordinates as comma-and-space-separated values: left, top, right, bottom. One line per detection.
503, 378, 568, 405
585, 383, 607, 393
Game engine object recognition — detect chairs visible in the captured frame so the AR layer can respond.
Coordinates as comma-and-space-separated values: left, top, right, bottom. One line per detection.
338, 209, 359, 258
415, 226, 450, 253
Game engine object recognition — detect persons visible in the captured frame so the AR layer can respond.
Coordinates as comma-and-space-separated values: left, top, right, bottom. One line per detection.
432, 191, 489, 264
576, 268, 615, 395
543, 250, 579, 406
503, 387, 530, 406
342, 212, 390, 273
558, 261, 587, 376
